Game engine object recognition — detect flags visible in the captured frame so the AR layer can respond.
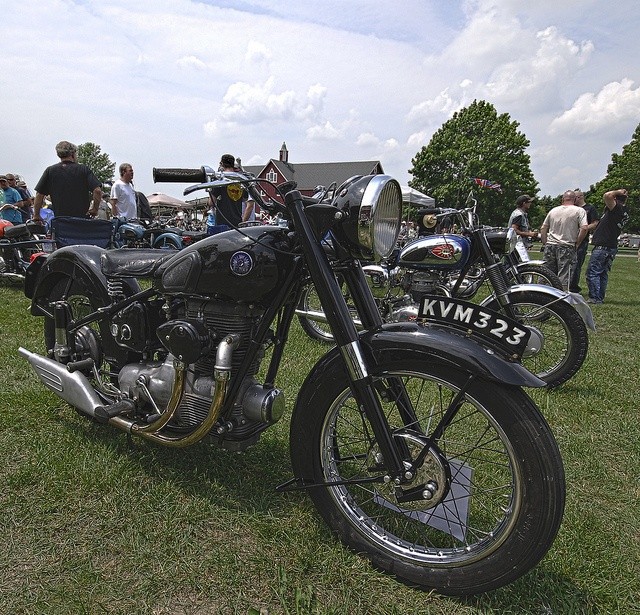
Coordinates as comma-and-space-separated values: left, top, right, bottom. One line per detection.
469, 176, 503, 194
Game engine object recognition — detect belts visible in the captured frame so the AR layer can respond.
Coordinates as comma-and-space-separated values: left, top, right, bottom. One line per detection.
596, 246, 617, 251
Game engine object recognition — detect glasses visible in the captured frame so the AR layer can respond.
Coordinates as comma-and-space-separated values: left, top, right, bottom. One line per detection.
7, 178, 14, 181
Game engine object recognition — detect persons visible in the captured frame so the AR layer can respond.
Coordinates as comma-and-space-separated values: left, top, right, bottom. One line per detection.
102, 192, 112, 220
535, 190, 589, 292
110, 162, 138, 219
397, 220, 407, 250
207, 153, 256, 227
585, 188, 629, 305
5, 174, 32, 223
0, 174, 24, 225
175, 209, 188, 229
30, 140, 103, 226
15, 175, 22, 185
46, 194, 51, 209
16, 181, 28, 193
232, 162, 257, 225
408, 220, 420, 234
569, 190, 598, 292
507, 194, 539, 262
88, 188, 106, 220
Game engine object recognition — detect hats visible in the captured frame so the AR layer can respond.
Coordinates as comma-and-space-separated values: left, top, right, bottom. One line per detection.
516, 194, 535, 207
220, 154, 234, 167
17, 181, 26, 187
0, 175, 7, 181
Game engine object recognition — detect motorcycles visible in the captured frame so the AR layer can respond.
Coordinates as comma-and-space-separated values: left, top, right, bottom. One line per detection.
18, 158, 566, 595
0, 202, 57, 280
334, 190, 563, 320
295, 206, 597, 389
111, 214, 192, 249
